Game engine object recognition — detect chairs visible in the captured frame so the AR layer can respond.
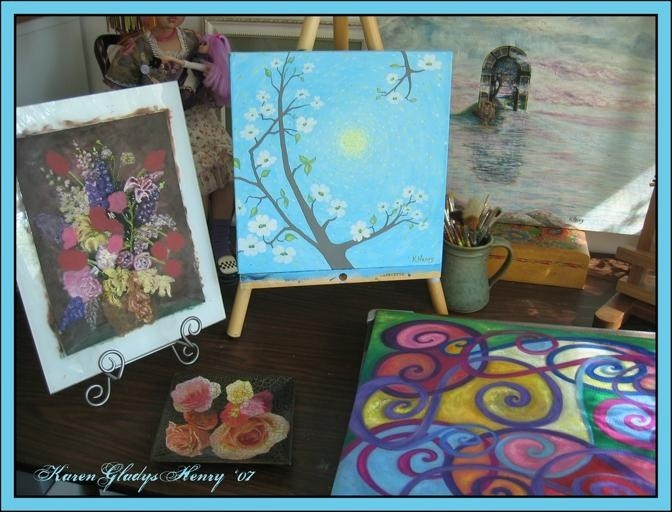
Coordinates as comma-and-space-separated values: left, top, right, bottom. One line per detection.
93, 34, 136, 77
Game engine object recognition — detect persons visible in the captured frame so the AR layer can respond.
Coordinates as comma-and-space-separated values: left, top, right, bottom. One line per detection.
103, 16, 238, 279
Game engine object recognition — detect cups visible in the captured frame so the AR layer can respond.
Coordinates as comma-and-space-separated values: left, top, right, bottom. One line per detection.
440, 232, 512, 315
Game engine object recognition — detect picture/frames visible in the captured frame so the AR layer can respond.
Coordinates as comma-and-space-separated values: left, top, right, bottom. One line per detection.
15, 81, 227, 397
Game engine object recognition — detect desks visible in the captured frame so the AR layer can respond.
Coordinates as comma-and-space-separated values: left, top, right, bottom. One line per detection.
14, 253, 655, 499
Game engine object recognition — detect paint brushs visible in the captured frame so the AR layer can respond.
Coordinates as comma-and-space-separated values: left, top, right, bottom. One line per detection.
443, 192, 502, 248
